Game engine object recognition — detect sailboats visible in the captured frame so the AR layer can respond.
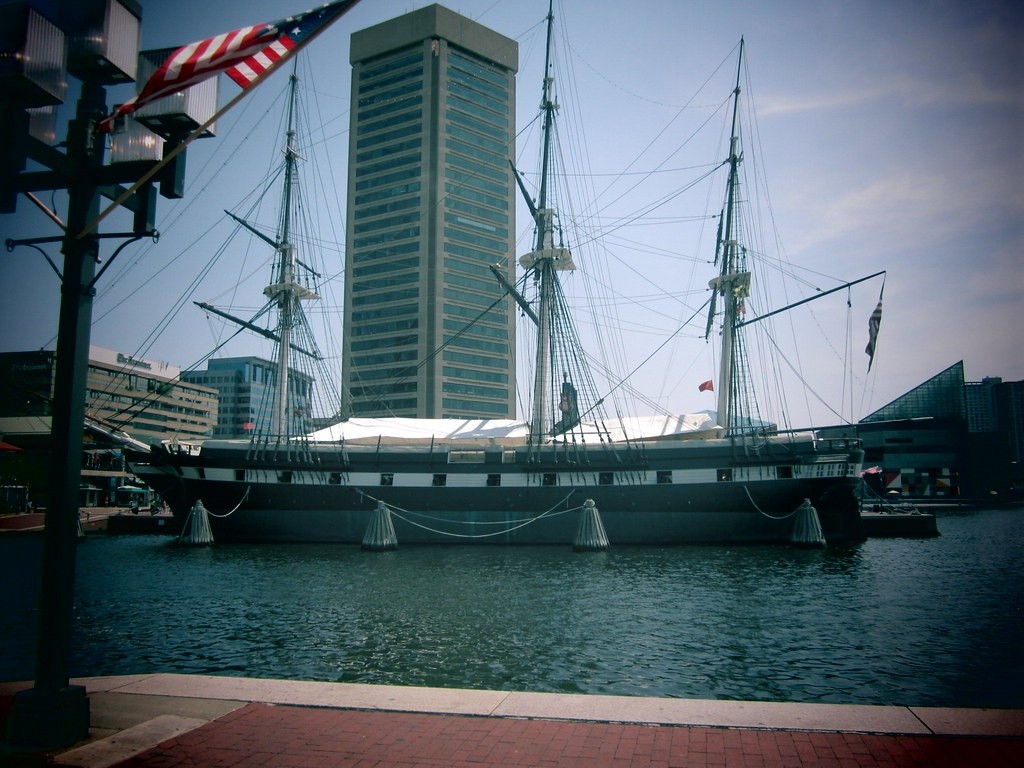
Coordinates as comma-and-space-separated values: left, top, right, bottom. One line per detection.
9, 0, 941, 550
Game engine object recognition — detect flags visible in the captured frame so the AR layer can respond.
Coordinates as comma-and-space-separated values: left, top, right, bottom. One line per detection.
699, 380, 714, 392
100, 0, 359, 125
864, 278, 887, 375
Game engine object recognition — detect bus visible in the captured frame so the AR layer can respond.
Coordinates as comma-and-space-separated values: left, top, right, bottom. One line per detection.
116, 485, 151, 511
